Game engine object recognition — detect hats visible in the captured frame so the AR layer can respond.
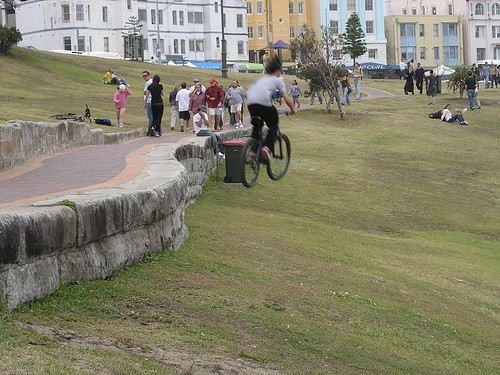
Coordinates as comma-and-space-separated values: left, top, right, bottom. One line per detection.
208, 78, 216, 84
199, 105, 207, 112
119, 84, 125, 91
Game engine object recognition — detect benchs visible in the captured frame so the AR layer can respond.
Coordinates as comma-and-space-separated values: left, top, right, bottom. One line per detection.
165, 54, 189, 66
236, 63, 251, 73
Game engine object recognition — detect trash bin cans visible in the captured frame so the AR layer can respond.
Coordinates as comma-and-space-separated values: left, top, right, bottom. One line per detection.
425, 75, 441, 94
222, 141, 247, 183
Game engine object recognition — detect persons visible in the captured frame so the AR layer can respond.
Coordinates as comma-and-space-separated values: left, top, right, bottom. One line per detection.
310, 62, 363, 106
101, 68, 130, 87
142, 71, 165, 137
274, 72, 303, 109
441, 105, 469, 125
170, 77, 247, 133
398, 58, 437, 105
193, 105, 226, 159
466, 61, 500, 110
113, 84, 131, 129
246, 53, 298, 165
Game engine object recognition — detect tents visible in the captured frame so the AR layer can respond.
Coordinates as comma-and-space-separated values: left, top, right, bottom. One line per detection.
345, 56, 408, 71
469, 59, 500, 66
425, 65, 456, 79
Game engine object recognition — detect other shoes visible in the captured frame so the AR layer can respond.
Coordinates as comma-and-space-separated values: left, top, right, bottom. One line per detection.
181, 125, 184, 132
218, 151, 224, 156
155, 132, 160, 136
262, 146, 272, 162
171, 127, 174, 129
235, 123, 239, 128
186, 128, 189, 132
219, 155, 225, 160
239, 123, 243, 128
151, 127, 154, 136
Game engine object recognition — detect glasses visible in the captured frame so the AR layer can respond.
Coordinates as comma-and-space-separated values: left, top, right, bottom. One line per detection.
143, 75, 148, 77
194, 81, 199, 83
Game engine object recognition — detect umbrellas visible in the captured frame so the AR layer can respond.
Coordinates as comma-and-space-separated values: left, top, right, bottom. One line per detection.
265, 40, 294, 62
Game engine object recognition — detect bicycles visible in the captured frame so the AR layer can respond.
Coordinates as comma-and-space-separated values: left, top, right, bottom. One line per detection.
50, 103, 93, 124
238, 109, 293, 187
